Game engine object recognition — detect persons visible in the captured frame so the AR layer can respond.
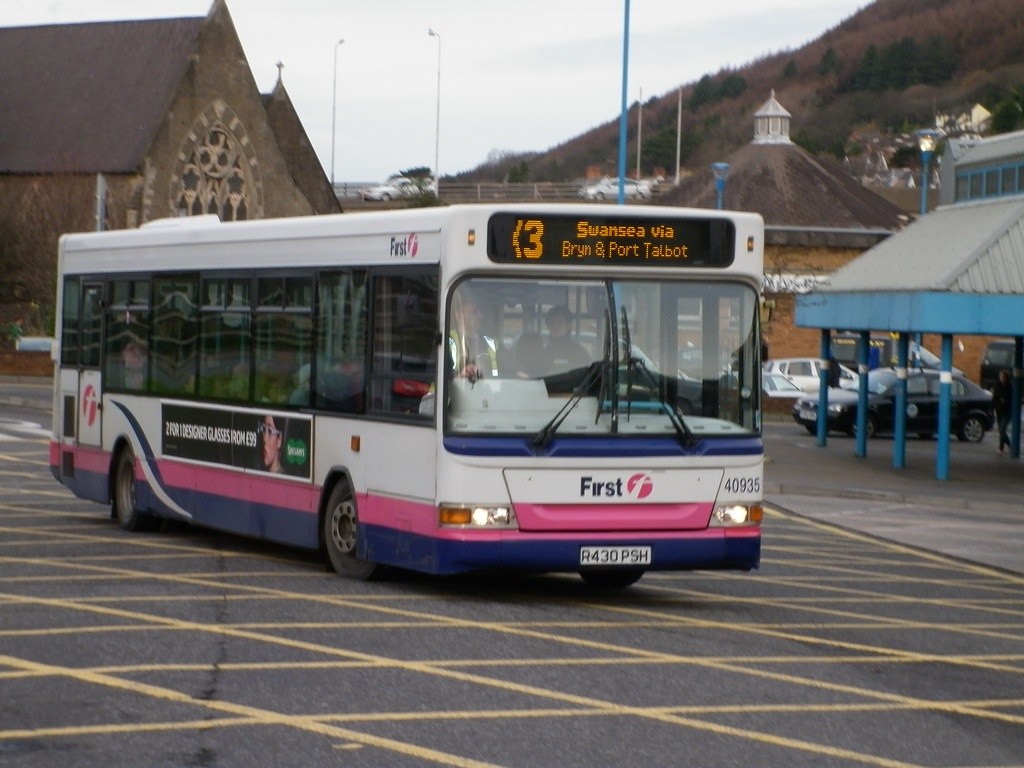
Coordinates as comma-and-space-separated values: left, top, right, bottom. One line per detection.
429, 300, 529, 394
259, 416, 292, 476
540, 302, 591, 391
178, 360, 346, 407
988, 369, 1014, 456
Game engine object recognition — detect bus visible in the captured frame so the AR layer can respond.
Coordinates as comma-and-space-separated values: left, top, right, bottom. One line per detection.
48, 203, 767, 596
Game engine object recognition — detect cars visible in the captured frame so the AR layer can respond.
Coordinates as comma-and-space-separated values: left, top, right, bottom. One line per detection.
792, 369, 995, 445
729, 371, 811, 399
515, 335, 658, 373
580, 176, 652, 201
764, 358, 888, 398
361, 178, 439, 203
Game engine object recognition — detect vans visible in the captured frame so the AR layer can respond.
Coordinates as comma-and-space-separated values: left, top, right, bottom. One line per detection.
979, 343, 1017, 397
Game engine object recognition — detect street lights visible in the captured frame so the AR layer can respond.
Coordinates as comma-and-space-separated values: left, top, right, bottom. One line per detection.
709, 161, 731, 210
426, 29, 442, 183
916, 128, 942, 214
330, 38, 345, 189
